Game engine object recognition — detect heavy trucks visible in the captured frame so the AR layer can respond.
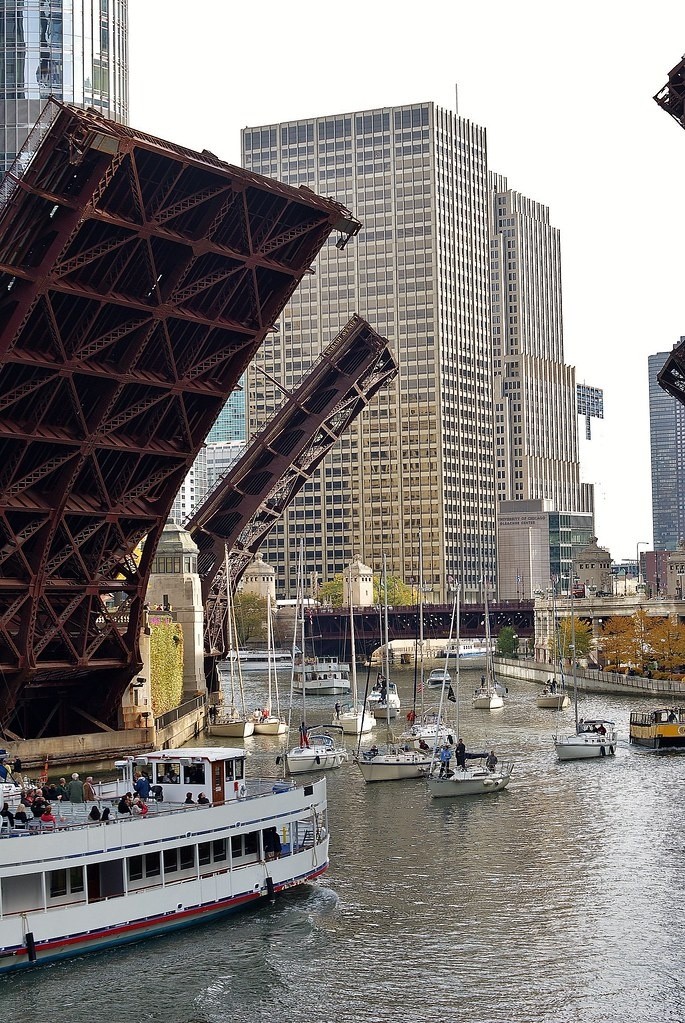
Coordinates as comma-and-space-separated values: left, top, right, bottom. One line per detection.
573, 583, 613, 598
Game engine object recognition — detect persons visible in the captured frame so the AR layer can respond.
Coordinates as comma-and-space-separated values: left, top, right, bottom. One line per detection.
407, 710, 415, 727
265, 827, 282, 862
549, 656, 652, 684
544, 678, 557, 694
372, 683, 387, 705
455, 739, 466, 772
439, 745, 452, 779
252, 708, 268, 723
486, 751, 498, 773
370, 744, 378, 755
667, 711, 677, 722
578, 719, 606, 736
144, 602, 173, 612
0, 754, 214, 838
299, 722, 311, 749
335, 700, 341, 720
481, 675, 485, 691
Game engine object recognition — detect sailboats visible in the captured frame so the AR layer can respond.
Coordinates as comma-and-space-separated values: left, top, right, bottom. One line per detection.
205, 538, 618, 797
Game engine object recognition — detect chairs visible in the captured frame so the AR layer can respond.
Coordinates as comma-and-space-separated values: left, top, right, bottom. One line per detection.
0, 800, 209, 838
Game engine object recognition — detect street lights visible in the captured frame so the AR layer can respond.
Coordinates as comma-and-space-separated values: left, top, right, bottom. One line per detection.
656, 549, 671, 596
637, 541, 649, 582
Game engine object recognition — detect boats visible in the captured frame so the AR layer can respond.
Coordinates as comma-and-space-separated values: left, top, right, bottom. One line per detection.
629, 707, 685, 749
0, 745, 331, 975
215, 648, 293, 671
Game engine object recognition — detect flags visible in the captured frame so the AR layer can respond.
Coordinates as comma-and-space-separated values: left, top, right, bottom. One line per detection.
417, 674, 424, 693
447, 685, 456, 703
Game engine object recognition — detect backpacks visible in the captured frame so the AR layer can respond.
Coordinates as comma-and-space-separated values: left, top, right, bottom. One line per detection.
140, 804, 148, 814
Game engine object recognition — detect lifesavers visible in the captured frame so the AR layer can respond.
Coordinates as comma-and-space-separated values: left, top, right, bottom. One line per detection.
677, 725, 685, 737
237, 784, 247, 799
